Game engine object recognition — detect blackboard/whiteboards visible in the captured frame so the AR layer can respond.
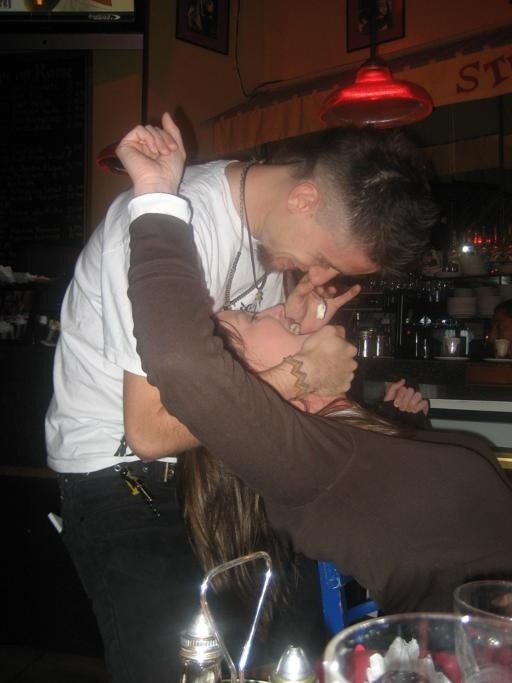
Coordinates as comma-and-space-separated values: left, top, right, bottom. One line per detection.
1, 50, 92, 246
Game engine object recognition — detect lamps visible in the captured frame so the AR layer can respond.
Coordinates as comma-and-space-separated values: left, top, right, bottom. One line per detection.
318, 1, 435, 128
97, 0, 150, 176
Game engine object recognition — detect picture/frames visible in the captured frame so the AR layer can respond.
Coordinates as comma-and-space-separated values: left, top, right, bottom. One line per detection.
172, 0, 230, 53
345, 0, 406, 52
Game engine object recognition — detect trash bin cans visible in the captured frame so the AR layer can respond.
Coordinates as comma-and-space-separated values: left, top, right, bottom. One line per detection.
1, 271, 61, 345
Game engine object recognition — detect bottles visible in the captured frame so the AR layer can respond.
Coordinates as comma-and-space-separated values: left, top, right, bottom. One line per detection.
444, 222, 512, 276
177, 610, 225, 683
348, 308, 432, 359
267, 642, 317, 683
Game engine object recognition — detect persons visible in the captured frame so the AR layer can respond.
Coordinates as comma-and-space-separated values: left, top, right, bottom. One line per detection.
484, 299, 511, 359
115, 111, 512, 624
40, 120, 446, 681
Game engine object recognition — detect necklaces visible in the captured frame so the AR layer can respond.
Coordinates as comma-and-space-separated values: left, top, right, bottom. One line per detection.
216, 158, 268, 312
235, 158, 270, 304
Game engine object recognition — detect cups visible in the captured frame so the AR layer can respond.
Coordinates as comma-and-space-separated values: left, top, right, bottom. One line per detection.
366, 273, 448, 303
321, 612, 511, 682
452, 580, 511, 683
495, 337, 510, 357
440, 335, 466, 356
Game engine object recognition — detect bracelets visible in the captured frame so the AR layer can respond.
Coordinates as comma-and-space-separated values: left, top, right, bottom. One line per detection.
283, 355, 314, 399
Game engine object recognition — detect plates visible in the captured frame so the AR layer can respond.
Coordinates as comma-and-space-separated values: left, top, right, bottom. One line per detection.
433, 356, 470, 361
445, 284, 511, 320
482, 358, 511, 363
